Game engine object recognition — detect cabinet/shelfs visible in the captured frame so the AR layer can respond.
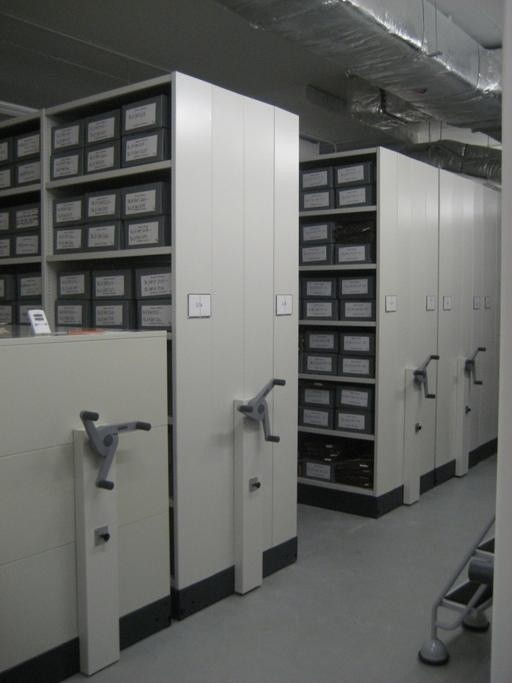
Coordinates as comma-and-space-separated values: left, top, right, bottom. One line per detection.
298, 146, 440, 507
0, 73, 300, 595
1, 329, 170, 674
441, 168, 498, 477
